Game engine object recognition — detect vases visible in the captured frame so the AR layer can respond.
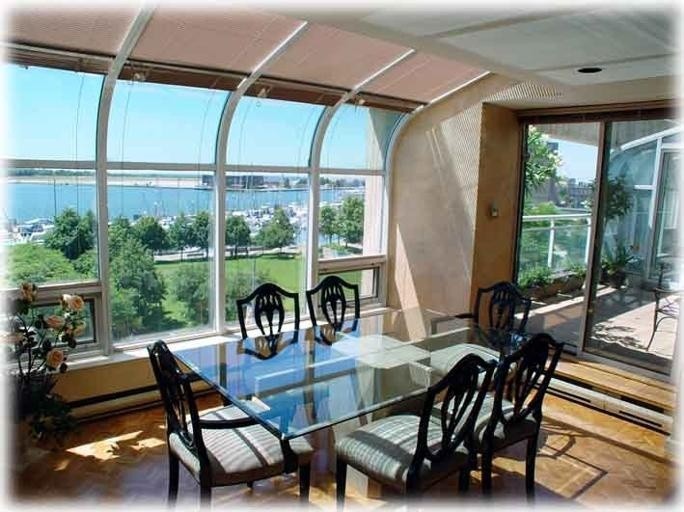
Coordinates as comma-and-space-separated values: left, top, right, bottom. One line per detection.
10, 414, 32, 442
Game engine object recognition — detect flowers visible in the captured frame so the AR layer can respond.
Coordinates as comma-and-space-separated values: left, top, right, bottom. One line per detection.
1, 279, 86, 449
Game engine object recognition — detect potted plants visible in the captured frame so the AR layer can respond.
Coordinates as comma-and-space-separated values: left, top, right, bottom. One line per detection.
598, 243, 635, 288
561, 261, 587, 291
